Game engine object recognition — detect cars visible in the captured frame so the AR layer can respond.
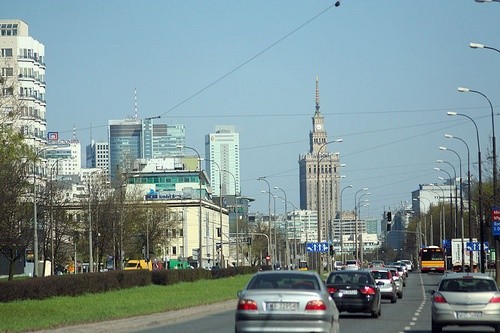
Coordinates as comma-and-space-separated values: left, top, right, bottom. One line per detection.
367, 270, 398, 303
431, 271, 500, 332
385, 269, 404, 298
384, 259, 413, 286
235, 270, 340, 333
326, 270, 382, 317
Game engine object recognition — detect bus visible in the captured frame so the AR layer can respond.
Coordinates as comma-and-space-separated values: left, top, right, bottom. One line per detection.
419, 245, 444, 273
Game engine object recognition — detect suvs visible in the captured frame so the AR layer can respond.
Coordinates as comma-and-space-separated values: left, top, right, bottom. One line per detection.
368, 261, 385, 265
345, 260, 360, 270
323, 260, 343, 270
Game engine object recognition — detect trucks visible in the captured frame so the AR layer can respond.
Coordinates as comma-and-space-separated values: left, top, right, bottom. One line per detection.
451, 237, 479, 270
124, 260, 152, 270
170, 259, 189, 269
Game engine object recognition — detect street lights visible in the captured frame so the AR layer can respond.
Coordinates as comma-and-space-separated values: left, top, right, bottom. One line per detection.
415, 159, 458, 244
439, 146, 465, 273
445, 134, 474, 273
198, 158, 224, 269
33, 145, 57, 277
176, 145, 202, 270
458, 87, 497, 206
446, 111, 486, 273
86, 167, 109, 271
215, 169, 239, 266
316, 138, 344, 277
325, 164, 371, 258
257, 176, 300, 262
